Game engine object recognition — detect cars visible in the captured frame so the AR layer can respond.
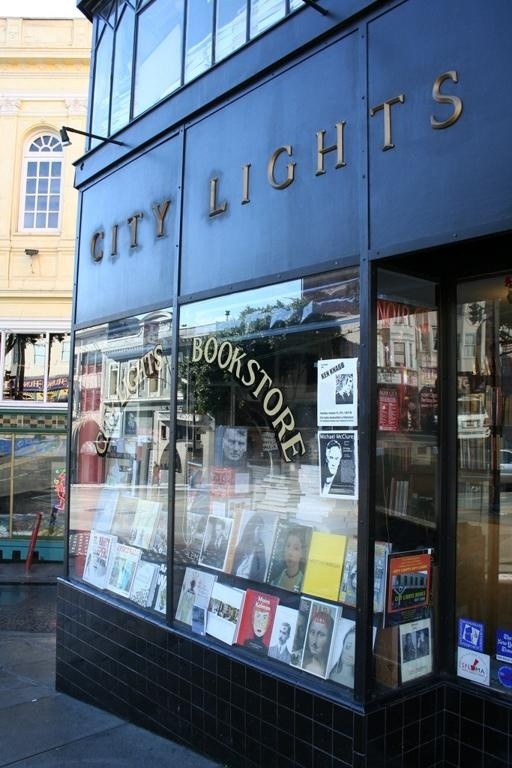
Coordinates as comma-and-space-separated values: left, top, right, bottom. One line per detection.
499, 447, 512, 488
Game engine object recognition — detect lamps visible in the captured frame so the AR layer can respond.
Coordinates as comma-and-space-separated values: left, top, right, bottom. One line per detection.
59, 126, 123, 164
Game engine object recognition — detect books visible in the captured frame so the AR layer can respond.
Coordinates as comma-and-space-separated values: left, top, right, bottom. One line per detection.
73, 303, 491, 696
